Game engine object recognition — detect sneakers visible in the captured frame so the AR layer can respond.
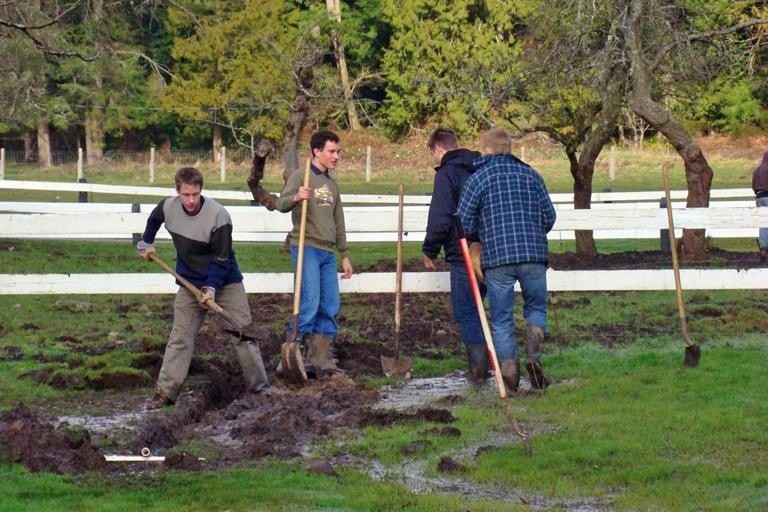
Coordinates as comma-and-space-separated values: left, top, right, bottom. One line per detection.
147, 392, 174, 411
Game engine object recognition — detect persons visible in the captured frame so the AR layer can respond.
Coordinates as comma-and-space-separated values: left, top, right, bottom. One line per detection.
420, 126, 557, 392
137, 168, 283, 411
751, 151, 768, 260
275, 131, 353, 377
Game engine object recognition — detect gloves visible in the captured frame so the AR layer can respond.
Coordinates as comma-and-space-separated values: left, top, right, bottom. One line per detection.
198, 286, 215, 309
137, 240, 157, 263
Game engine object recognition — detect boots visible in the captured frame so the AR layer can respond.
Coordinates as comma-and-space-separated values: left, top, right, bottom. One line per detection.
306, 332, 348, 381
499, 322, 548, 392
459, 340, 488, 383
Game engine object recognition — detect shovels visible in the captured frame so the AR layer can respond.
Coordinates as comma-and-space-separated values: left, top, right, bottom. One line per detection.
281, 158, 311, 385
381, 183, 413, 378
147, 252, 267, 339
452, 213, 533, 456
662, 165, 702, 367
